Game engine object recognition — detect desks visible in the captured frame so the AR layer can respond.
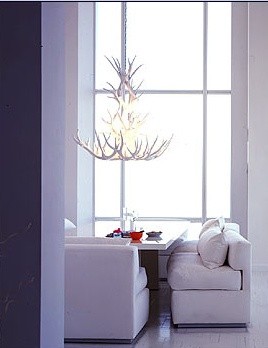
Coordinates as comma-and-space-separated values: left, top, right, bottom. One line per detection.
64, 221, 191, 291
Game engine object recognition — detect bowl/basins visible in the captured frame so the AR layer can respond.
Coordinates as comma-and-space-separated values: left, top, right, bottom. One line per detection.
146, 237, 162, 241
130, 240, 142, 244
129, 232, 143, 240
146, 231, 162, 237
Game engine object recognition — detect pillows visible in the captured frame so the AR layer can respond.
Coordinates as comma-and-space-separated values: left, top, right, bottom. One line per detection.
197, 226, 228, 269
198, 215, 224, 239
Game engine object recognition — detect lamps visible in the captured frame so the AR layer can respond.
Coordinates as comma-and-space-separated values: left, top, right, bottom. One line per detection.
73, 2, 175, 161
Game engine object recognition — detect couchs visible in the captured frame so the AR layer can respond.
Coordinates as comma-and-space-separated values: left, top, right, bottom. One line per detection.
166, 222, 251, 329
64, 244, 150, 343
